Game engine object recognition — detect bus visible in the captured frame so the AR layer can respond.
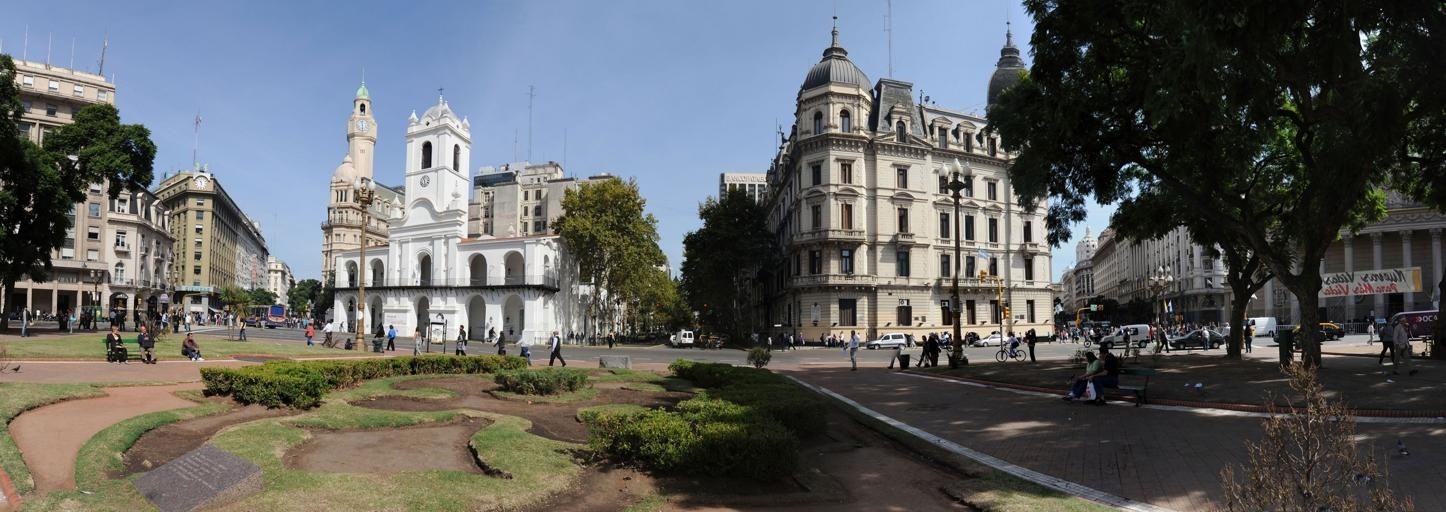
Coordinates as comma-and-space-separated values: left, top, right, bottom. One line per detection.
241, 303, 289, 330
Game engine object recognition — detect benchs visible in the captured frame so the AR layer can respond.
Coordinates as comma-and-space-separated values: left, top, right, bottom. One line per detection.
1072, 366, 1153, 408
101, 337, 152, 360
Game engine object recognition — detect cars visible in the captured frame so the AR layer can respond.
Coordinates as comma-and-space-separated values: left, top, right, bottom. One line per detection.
1169, 329, 1225, 350
1320, 323, 1348, 340
633, 327, 669, 344
866, 332, 910, 350
971, 334, 1013, 347
1274, 325, 1327, 344
668, 334, 676, 344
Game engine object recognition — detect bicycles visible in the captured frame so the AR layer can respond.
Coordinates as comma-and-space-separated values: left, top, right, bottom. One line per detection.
994, 340, 1028, 362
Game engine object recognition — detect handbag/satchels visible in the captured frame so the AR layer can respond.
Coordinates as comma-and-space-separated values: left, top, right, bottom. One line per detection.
1086, 380, 1096, 400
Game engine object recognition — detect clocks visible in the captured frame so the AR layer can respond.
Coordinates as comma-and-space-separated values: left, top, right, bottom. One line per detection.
356, 119, 370, 132
419, 174, 432, 187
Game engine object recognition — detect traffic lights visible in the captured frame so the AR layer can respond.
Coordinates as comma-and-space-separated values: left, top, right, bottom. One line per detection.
979, 270, 986, 284
703, 303, 708, 313
1098, 305, 1103, 311
1005, 307, 1009, 318
656, 303, 662, 311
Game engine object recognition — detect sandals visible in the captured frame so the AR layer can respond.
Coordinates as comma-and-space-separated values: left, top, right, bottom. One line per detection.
1062, 392, 1075, 401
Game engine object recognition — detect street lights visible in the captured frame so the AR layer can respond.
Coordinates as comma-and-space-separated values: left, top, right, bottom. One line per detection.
349, 175, 377, 353
1158, 264, 1174, 353
1144, 277, 1167, 354
773, 322, 786, 353
89, 266, 103, 331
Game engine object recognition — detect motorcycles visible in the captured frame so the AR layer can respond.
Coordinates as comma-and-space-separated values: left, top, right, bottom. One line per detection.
1083, 331, 1109, 348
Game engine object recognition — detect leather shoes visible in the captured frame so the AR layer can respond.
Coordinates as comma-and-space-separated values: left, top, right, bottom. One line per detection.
851, 347, 857, 349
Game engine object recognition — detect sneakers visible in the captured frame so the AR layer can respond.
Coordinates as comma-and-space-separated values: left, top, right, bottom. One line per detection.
1084, 398, 1106, 406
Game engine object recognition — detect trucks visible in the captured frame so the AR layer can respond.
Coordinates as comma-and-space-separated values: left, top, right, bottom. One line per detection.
672, 331, 696, 348
1097, 324, 1152, 350
1243, 317, 1280, 338
1379, 309, 1441, 341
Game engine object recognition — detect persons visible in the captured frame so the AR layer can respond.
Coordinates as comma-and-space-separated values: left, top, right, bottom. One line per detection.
181, 333, 206, 361
548, 330, 566, 367
493, 331, 506, 355
286, 316, 397, 354
704, 329, 954, 371
18, 305, 267, 342
1004, 316, 1252, 405
565, 329, 639, 349
459, 325, 466, 341
515, 329, 533, 368
488, 327, 497, 342
412, 326, 423, 357
455, 335, 467, 357
106, 325, 128, 365
137, 326, 157, 364
1366, 318, 1418, 377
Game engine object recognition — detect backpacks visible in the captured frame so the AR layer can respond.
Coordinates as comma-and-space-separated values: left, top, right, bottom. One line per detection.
141, 334, 154, 348
